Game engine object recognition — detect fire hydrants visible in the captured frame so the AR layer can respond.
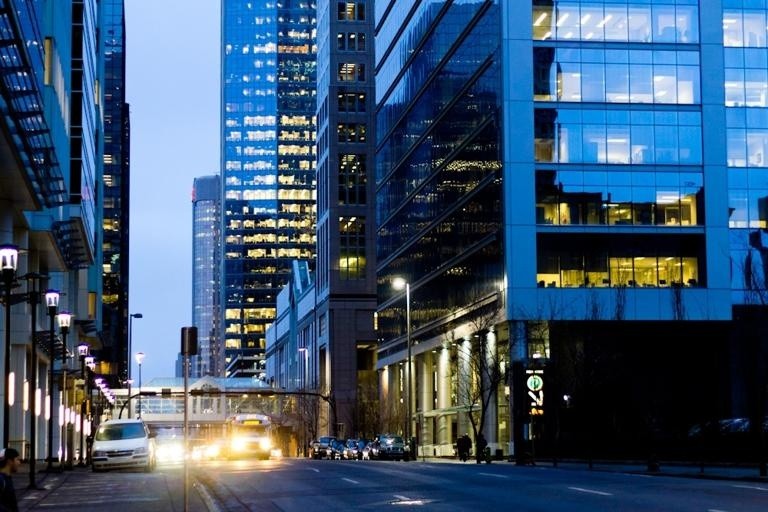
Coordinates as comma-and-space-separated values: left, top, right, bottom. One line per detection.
484, 446, 492, 464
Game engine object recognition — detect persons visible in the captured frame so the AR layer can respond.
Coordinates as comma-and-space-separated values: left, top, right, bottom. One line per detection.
0, 447, 21, 512
456, 432, 487, 464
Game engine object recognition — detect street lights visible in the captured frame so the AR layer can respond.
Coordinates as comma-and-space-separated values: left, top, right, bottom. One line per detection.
126, 312, 142, 419
55, 307, 75, 473
1, 241, 22, 490
20, 271, 51, 491
75, 342, 117, 464
294, 345, 309, 396
136, 353, 145, 417
41, 287, 66, 474
388, 274, 413, 454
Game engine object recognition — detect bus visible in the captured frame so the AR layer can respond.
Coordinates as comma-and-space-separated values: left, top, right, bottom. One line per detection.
220, 412, 275, 460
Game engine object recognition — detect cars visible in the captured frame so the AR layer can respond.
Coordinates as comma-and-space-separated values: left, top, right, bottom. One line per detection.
680, 414, 768, 434
86, 416, 158, 473
307, 429, 415, 463
